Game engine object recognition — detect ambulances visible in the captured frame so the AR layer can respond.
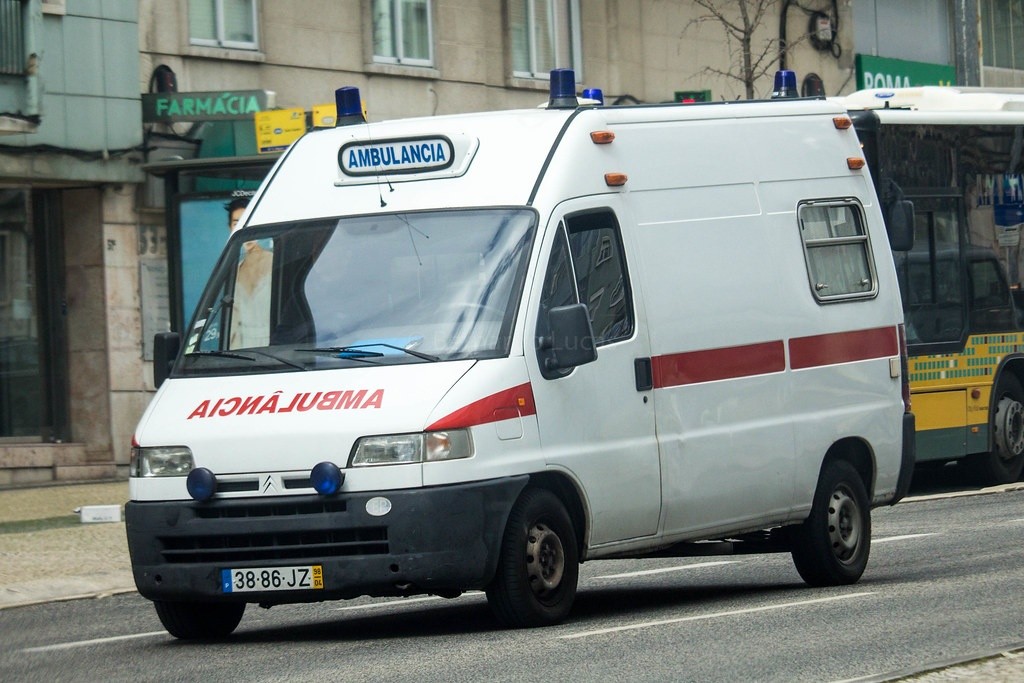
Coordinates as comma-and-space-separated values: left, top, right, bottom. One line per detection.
122, 67, 917, 642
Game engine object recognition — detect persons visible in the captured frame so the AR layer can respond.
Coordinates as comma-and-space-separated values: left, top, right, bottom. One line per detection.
223, 197, 273, 350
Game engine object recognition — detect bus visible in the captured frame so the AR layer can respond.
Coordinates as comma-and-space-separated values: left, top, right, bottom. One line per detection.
839, 84, 1023, 484
